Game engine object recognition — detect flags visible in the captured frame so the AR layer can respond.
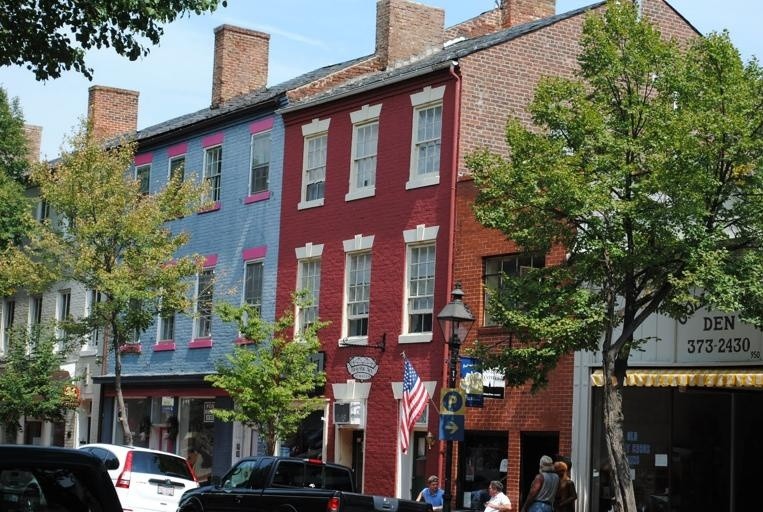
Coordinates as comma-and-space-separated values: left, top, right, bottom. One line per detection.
398, 359, 430, 455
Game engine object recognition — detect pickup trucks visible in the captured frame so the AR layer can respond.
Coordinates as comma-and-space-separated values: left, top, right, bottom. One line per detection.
176, 454, 434, 512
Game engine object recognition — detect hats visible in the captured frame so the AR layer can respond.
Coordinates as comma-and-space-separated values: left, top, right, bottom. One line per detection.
428, 475, 439, 484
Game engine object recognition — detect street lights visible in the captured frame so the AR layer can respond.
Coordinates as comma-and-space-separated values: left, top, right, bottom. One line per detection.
435, 279, 478, 512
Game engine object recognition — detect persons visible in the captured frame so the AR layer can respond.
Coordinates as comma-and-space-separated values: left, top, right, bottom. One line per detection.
518, 455, 559, 512
483, 481, 511, 512
415, 476, 444, 512
551, 462, 576, 512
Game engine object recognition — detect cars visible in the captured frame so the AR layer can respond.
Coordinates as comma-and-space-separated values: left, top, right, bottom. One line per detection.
77, 442, 200, 512
0, 443, 124, 512
